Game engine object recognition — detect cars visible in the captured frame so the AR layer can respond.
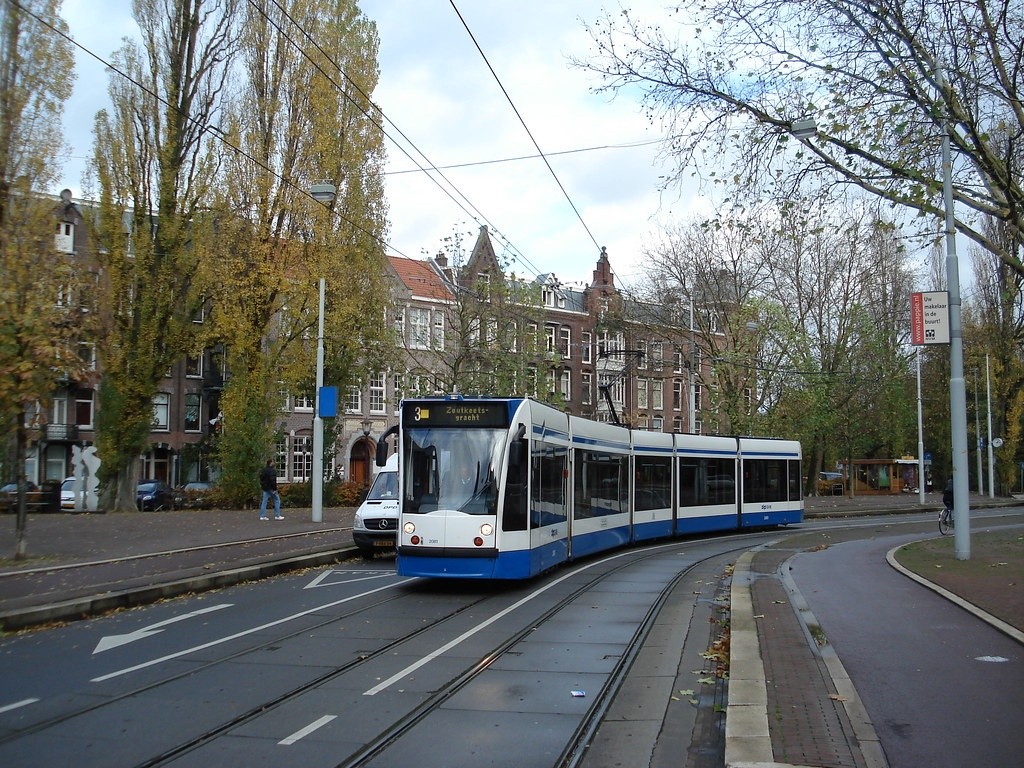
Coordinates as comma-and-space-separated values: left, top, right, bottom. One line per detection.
0, 476, 218, 513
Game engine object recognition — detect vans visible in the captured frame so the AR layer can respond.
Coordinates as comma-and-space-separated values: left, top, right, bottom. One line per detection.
819, 471, 843, 496
351, 450, 451, 555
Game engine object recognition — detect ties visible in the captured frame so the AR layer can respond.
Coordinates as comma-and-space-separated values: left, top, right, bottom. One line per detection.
464, 480, 468, 484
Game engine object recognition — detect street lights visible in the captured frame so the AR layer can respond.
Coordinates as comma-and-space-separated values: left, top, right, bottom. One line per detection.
790, 58, 971, 561
308, 177, 338, 522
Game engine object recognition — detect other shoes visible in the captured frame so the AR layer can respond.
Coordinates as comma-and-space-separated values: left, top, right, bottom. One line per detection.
274, 516, 285, 520
260, 516, 270, 520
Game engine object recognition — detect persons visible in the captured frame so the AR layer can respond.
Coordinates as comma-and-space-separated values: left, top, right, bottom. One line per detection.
924, 474, 933, 495
259, 459, 285, 521
942, 479, 954, 523
453, 464, 474, 492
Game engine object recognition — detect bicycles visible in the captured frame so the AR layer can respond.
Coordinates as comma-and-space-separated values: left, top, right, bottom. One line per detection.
938, 501, 954, 535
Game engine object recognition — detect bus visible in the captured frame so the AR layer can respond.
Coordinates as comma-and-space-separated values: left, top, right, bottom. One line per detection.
376, 350, 803, 579
